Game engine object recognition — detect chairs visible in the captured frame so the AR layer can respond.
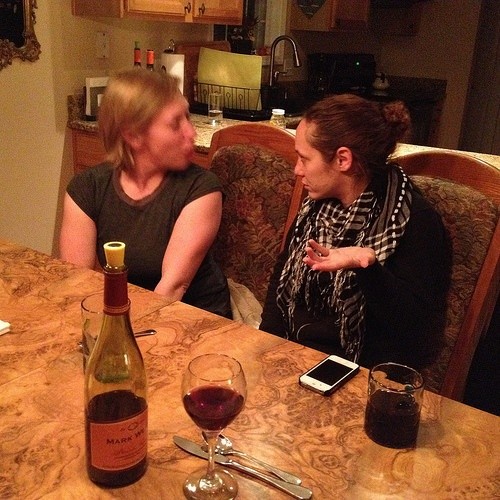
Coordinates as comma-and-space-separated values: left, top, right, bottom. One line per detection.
208, 123, 307, 309
389, 148, 500, 404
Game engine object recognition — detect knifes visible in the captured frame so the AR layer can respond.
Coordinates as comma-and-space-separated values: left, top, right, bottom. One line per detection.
172, 434, 312, 500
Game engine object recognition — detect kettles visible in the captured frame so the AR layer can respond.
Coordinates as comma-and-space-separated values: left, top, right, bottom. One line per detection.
370, 71, 389, 90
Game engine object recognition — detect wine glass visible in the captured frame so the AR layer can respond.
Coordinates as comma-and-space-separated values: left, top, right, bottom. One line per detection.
180, 352, 248, 500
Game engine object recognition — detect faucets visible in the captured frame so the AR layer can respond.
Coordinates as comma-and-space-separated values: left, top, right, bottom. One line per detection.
268, 34, 303, 87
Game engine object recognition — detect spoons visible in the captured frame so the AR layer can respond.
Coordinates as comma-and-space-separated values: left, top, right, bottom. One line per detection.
200, 430, 303, 485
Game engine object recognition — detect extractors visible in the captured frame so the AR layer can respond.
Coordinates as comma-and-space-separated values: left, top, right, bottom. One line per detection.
335, 18, 417, 40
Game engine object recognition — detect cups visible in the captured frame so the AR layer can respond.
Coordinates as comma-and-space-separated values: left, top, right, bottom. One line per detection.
364, 362, 425, 450
81, 292, 131, 376
208, 92, 224, 128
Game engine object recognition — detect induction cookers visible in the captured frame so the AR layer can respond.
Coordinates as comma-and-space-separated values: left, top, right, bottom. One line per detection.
307, 53, 407, 105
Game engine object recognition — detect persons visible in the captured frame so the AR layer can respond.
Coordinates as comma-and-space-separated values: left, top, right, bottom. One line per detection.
59, 68, 231, 319
259, 93, 449, 373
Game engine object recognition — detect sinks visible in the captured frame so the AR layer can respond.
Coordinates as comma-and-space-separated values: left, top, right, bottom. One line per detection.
271, 86, 318, 118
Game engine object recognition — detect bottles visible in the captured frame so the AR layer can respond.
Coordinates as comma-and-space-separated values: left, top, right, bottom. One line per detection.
146, 48, 156, 73
269, 109, 286, 131
83, 242, 149, 489
133, 39, 142, 71
258, 46, 271, 55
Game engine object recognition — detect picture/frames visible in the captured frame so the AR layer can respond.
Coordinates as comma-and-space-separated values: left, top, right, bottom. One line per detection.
0, 0, 42, 71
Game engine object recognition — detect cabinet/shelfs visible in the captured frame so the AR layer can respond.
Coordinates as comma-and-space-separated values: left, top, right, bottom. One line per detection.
71, 0, 244, 26
290, 0, 374, 33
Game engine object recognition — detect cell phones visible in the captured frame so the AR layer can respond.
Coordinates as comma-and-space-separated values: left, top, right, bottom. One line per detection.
299, 355, 359, 397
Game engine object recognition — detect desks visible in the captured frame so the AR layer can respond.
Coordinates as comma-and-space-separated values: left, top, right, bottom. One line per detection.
0, 240, 500, 500
66, 112, 500, 178
